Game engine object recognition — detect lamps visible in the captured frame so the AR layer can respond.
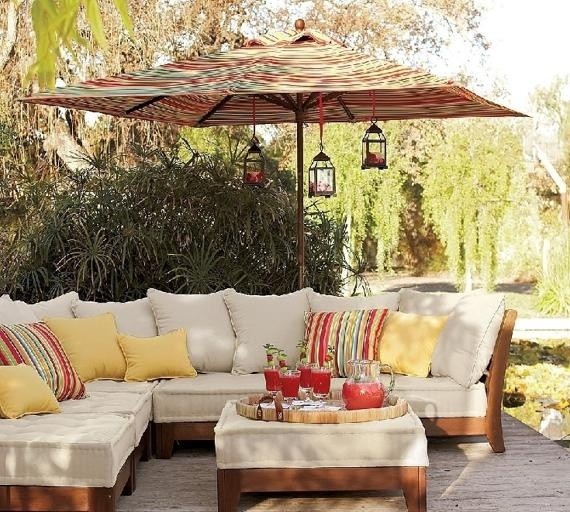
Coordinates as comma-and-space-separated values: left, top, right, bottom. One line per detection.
241, 91, 389, 199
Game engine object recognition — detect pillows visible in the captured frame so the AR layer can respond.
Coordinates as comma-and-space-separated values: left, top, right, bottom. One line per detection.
304, 310, 388, 376
304, 287, 401, 313
400, 287, 460, 316
379, 309, 446, 380
432, 293, 504, 389
71, 297, 159, 341
147, 286, 237, 376
30, 290, 80, 320
0, 362, 61, 420
42, 313, 126, 384
3, 321, 91, 402
223, 288, 310, 377
115, 328, 196, 380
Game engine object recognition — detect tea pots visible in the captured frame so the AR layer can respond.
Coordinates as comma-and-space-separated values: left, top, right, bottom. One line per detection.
342, 360, 395, 411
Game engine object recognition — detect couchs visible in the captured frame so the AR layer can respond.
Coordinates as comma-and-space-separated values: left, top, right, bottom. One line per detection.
0, 307, 517, 512
152, 286, 515, 454
1, 289, 159, 512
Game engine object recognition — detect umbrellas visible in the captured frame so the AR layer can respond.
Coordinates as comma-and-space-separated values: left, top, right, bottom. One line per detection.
14, 17, 534, 291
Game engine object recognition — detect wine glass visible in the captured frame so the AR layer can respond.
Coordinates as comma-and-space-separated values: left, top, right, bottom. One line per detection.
263, 363, 331, 410
